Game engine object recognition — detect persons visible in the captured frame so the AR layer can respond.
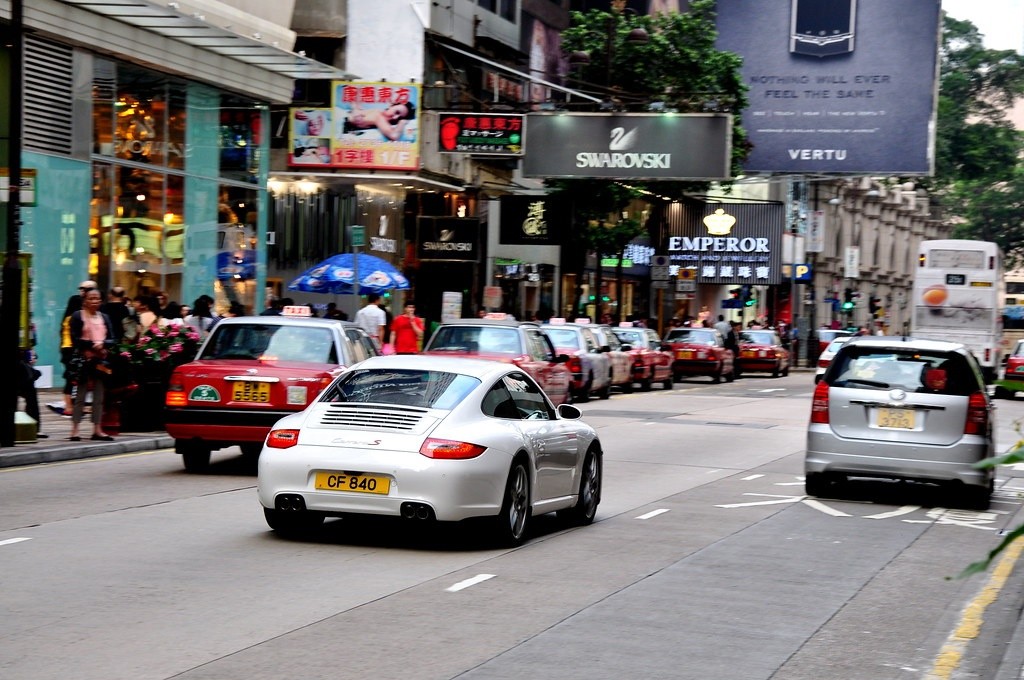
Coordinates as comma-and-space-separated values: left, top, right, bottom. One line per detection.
294, 111, 328, 157
269, 294, 425, 355
59, 280, 245, 441
480, 306, 554, 324
844, 321, 890, 336
602, 305, 797, 376
344, 101, 415, 141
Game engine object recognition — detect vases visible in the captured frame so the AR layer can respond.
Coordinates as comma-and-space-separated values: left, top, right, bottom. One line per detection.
115, 367, 166, 432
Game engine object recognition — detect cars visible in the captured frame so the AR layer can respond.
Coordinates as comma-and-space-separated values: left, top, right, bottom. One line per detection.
662, 327, 736, 384
161, 305, 384, 472
806, 329, 855, 363
520, 316, 675, 402
814, 336, 854, 384
419, 311, 576, 417
805, 334, 1014, 510
1001, 338, 1024, 380
736, 330, 791, 377
1002, 306, 1024, 330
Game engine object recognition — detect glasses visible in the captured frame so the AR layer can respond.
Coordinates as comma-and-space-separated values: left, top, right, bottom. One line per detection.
78, 287, 89, 292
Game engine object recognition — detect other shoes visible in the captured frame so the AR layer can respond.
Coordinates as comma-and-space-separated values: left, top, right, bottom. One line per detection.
92, 432, 113, 441
71, 437, 80, 441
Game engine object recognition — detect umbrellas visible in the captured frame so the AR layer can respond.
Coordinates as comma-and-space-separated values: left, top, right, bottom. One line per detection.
209, 249, 256, 281
287, 252, 410, 311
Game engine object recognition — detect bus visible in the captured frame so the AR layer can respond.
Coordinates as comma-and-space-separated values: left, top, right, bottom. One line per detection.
911, 238, 1005, 385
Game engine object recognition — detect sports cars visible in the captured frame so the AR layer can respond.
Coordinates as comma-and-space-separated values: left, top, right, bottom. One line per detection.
256, 353, 604, 549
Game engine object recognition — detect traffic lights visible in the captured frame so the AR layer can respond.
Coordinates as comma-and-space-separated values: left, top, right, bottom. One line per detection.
844, 289, 859, 313
868, 296, 882, 314
742, 285, 755, 308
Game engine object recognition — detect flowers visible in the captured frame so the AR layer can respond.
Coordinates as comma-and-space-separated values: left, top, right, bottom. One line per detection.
109, 324, 205, 366
